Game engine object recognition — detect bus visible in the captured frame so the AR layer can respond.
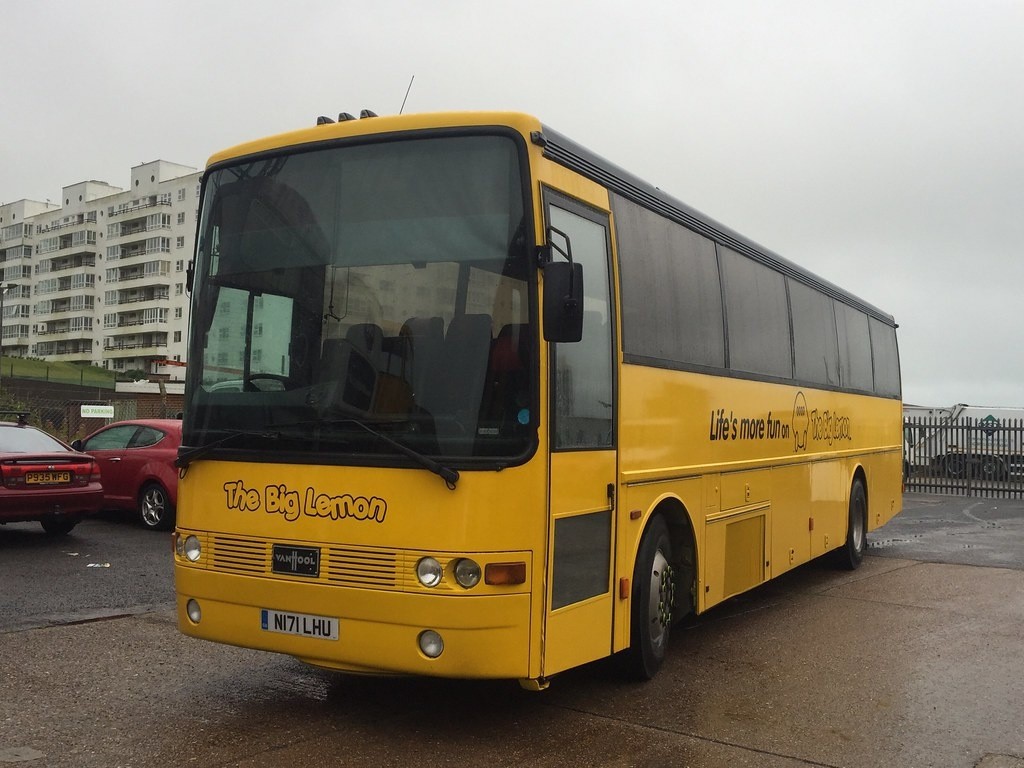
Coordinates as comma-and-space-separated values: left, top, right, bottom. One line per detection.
168, 110, 904, 694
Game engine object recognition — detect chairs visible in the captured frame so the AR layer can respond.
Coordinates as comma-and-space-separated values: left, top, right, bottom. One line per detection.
315, 313, 530, 408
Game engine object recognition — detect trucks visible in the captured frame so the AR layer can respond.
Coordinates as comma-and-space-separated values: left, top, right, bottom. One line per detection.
901, 400, 1024, 483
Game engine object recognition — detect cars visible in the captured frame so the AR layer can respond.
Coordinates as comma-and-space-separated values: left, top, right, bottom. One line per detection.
0, 419, 105, 538
67, 417, 183, 530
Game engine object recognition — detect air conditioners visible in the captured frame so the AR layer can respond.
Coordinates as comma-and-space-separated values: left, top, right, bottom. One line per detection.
102, 337, 114, 346
37, 323, 47, 332
103, 359, 113, 370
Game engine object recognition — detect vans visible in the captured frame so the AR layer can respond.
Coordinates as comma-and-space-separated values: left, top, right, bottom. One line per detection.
155, 379, 243, 442
